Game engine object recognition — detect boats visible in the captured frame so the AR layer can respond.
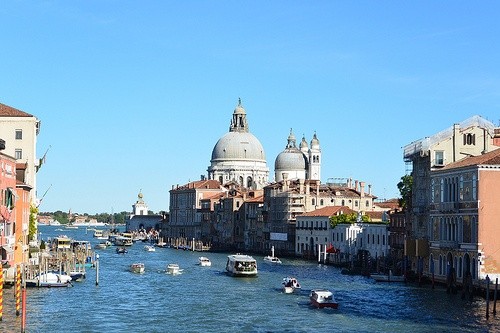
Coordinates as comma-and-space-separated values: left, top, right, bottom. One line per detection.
225, 254, 258, 277
198, 256, 211, 267
263, 245, 282, 264
94, 242, 107, 250
54, 228, 64, 232
34, 273, 72, 287
116, 247, 128, 254
166, 263, 180, 274
144, 245, 156, 252
370, 272, 405, 282
52, 237, 71, 251
281, 277, 301, 294
72, 240, 92, 253
129, 263, 145, 274
65, 225, 78, 230
309, 288, 339, 309
93, 228, 211, 253
69, 269, 86, 281
